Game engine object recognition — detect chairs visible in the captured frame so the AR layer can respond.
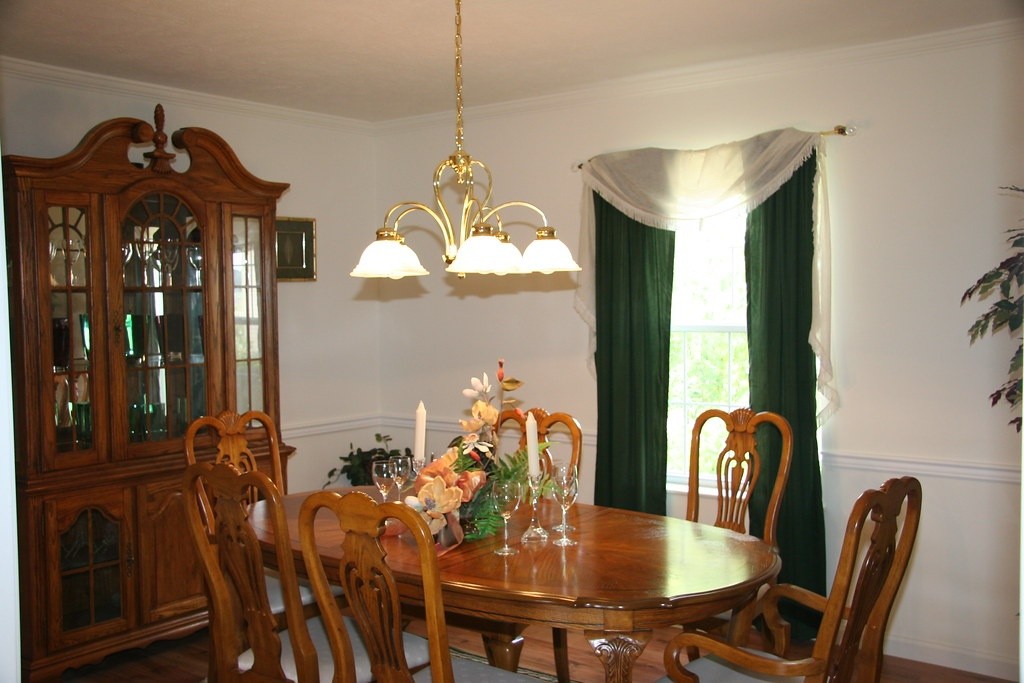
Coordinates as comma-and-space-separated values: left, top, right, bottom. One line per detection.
299, 491, 544, 683
184, 409, 346, 632
655, 476, 922, 683
686, 408, 793, 631
492, 408, 582, 505
183, 461, 431, 682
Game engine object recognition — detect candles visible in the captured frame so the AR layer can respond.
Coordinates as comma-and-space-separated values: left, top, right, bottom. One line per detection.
413, 399, 428, 459
524, 411, 541, 477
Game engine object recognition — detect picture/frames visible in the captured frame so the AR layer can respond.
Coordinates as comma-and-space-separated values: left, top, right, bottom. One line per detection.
275, 216, 317, 283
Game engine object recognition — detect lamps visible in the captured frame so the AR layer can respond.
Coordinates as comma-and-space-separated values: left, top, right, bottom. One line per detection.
350, 0, 582, 280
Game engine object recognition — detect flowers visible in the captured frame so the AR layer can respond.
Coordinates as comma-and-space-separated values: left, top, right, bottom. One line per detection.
403, 358, 563, 538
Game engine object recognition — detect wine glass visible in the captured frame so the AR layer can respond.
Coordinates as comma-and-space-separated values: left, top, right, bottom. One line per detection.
49, 239, 56, 286
120, 239, 202, 286
492, 480, 520, 556
553, 477, 577, 546
553, 463, 579, 532
62, 240, 79, 285
389, 455, 410, 504
373, 460, 397, 504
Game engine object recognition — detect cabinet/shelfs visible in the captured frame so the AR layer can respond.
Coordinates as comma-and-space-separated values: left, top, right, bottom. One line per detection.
0, 101, 297, 683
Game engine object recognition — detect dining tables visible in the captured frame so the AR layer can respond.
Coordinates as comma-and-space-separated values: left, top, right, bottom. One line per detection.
246, 485, 783, 683
